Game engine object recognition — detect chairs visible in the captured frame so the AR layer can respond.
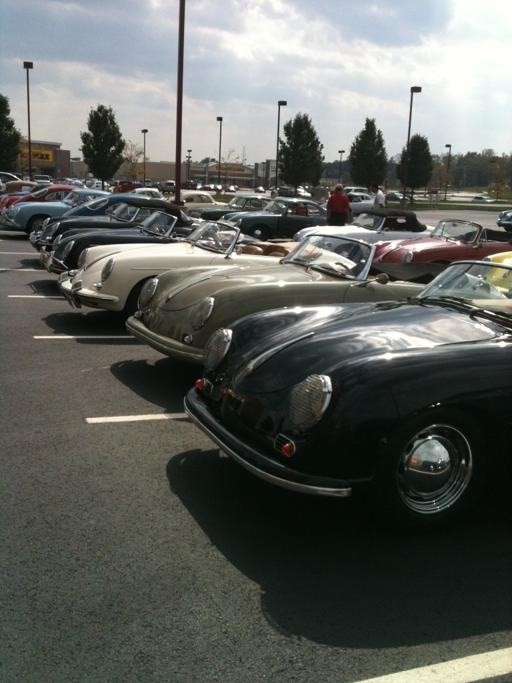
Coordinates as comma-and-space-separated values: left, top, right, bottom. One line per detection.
239, 241, 290, 257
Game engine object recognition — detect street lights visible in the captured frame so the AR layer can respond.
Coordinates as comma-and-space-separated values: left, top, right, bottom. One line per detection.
403, 85, 422, 190
20, 60, 35, 175
275, 100, 288, 182
443, 142, 452, 203
215, 115, 225, 184
337, 149, 347, 182
141, 129, 149, 184
184, 149, 193, 177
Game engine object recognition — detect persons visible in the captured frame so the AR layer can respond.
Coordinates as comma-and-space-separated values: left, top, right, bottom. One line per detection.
370, 183, 387, 207
112, 179, 121, 193
326, 183, 352, 225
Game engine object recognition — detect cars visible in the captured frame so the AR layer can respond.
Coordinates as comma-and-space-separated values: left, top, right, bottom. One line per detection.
496, 209, 512, 232
470, 195, 498, 203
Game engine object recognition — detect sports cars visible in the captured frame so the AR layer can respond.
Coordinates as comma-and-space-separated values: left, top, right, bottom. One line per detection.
291, 207, 448, 260
55, 219, 353, 317
124, 233, 512, 365
0, 171, 407, 278
183, 258, 510, 518
362, 218, 511, 284
